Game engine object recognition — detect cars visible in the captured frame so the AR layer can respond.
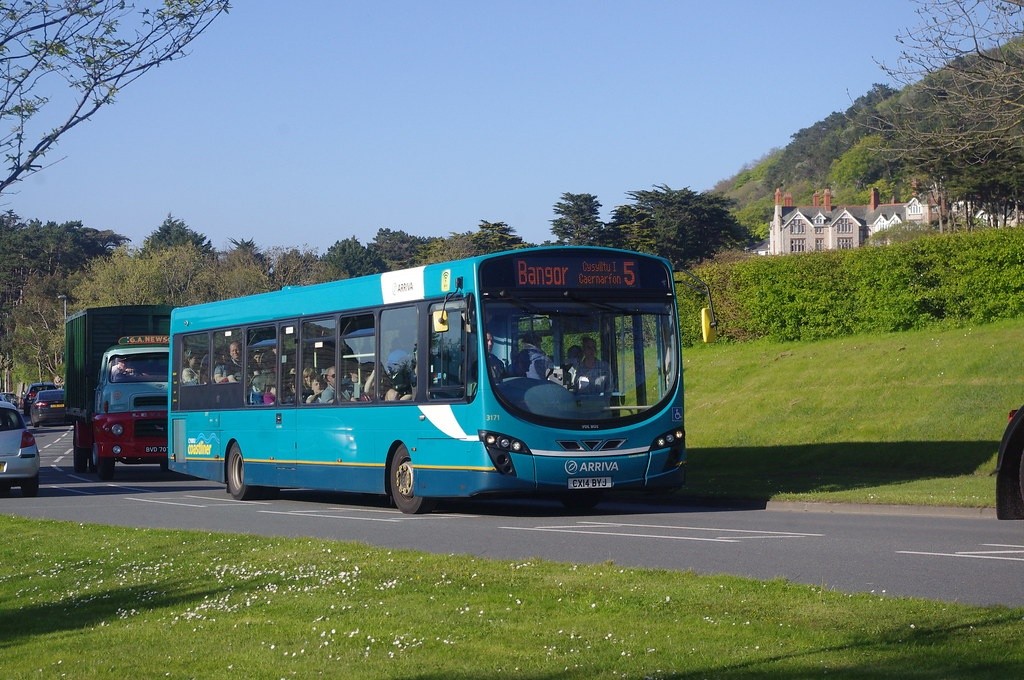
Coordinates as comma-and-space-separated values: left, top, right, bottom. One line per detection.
31, 389, 72, 427
0, 402, 40, 497
22, 383, 58, 416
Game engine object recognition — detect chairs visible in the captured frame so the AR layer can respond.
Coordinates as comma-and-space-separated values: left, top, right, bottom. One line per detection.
0, 414, 14, 430
182, 352, 467, 403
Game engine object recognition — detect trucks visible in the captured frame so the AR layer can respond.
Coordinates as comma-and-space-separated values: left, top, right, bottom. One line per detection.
64, 305, 184, 481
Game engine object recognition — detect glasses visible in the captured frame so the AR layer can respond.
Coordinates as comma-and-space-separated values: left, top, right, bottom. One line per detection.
327, 374, 335, 378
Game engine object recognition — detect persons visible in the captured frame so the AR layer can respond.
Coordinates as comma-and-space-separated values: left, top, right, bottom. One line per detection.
111, 357, 136, 380
181, 347, 208, 385
214, 342, 418, 404
470, 328, 504, 385
512, 331, 554, 380
568, 336, 612, 409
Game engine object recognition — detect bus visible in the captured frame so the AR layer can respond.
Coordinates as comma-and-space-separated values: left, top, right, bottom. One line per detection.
166, 245, 717, 515
